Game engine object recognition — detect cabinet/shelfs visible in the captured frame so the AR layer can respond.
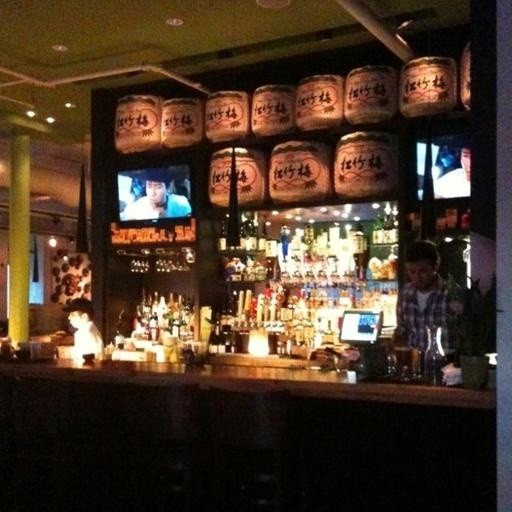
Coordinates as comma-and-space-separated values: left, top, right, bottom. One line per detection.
90, 23, 496, 369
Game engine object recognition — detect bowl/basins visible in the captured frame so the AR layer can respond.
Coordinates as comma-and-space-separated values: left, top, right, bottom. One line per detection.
82, 353, 95, 363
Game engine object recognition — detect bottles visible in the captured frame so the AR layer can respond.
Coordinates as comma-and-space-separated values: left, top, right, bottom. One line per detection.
422, 325, 448, 386
220, 202, 399, 281
184, 344, 194, 365
132, 288, 195, 342
208, 286, 397, 359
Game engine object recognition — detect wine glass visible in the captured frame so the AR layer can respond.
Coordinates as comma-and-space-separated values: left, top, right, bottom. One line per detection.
129, 249, 195, 273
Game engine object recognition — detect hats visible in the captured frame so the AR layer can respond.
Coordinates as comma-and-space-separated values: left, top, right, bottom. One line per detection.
62, 297, 95, 312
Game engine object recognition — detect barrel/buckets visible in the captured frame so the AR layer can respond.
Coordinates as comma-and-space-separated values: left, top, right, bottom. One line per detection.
208, 146, 266, 207
114, 95, 161, 154
268, 141, 332, 201
158, 99, 202, 150
341, 65, 394, 126
248, 85, 296, 138
293, 74, 345, 132
332, 129, 393, 199
398, 55, 459, 120
204, 91, 250, 144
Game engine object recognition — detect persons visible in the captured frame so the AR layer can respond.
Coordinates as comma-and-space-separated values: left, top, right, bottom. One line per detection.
418, 146, 472, 200
63, 298, 104, 354
122, 167, 192, 220
393, 240, 468, 377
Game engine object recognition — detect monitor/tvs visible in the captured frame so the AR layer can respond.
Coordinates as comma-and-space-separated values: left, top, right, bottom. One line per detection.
339, 310, 384, 345
111, 159, 197, 227
406, 132, 484, 206
